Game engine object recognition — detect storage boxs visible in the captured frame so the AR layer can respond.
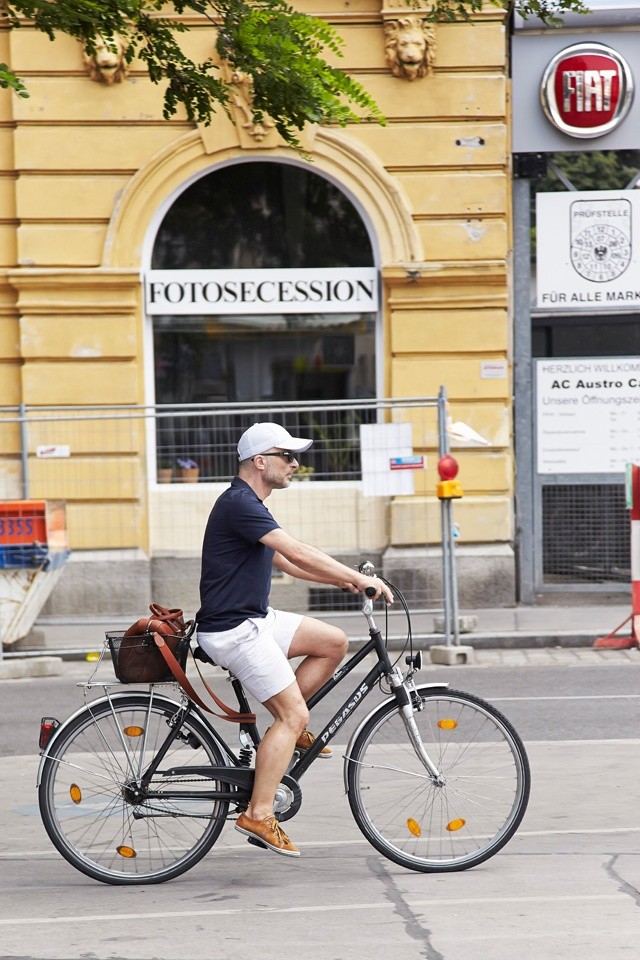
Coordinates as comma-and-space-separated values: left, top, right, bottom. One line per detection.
0, 500, 69, 570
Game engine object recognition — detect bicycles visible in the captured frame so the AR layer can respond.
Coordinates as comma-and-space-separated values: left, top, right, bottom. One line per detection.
36, 560, 531, 884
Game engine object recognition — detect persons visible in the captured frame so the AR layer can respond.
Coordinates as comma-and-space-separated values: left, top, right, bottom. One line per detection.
195, 422, 395, 858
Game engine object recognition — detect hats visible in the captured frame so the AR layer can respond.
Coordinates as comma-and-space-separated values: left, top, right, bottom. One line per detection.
237, 422, 313, 462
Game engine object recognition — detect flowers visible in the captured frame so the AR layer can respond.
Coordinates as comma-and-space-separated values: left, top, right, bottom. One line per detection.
292, 466, 314, 477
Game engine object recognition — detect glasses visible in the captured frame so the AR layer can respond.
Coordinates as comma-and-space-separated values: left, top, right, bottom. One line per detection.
251, 451, 298, 463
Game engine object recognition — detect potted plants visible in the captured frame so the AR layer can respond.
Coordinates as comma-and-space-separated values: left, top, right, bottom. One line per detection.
158, 460, 172, 483
178, 458, 199, 484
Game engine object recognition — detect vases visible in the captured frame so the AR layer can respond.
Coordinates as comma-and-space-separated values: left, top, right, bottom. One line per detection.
290, 478, 300, 481
302, 477, 311, 481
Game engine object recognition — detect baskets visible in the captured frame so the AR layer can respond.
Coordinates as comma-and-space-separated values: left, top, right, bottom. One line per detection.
105, 630, 192, 684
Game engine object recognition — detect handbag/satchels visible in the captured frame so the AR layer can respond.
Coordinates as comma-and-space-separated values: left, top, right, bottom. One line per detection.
117, 604, 184, 683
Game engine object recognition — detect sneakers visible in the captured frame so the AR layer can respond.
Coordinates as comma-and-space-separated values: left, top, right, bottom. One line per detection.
264, 726, 332, 758
234, 811, 301, 855
